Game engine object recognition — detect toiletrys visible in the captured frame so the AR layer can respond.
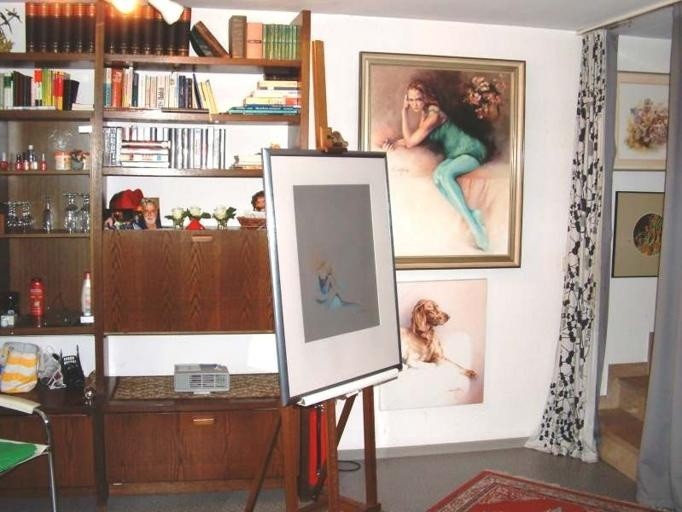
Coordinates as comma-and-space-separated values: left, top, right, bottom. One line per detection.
0, 140, 52, 176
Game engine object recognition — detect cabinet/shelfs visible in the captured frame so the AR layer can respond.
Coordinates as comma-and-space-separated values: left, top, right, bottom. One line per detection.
0, 0, 100, 498
98, 0, 311, 496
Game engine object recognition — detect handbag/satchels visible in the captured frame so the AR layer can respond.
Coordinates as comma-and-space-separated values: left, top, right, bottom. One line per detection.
59, 345, 84, 386
0, 341, 41, 394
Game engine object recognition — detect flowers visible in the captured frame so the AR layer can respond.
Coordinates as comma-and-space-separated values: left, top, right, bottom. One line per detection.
183, 205, 212, 219
163, 206, 187, 227
213, 206, 237, 227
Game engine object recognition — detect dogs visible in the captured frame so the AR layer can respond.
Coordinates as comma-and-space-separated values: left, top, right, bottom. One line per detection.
400, 299, 477, 379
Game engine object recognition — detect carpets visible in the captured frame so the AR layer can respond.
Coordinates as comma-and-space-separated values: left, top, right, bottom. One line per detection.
425, 470, 682, 512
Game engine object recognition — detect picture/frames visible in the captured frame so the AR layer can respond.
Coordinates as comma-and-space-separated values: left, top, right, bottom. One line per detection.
261, 149, 402, 407
611, 191, 665, 277
358, 52, 526, 270
613, 70, 670, 171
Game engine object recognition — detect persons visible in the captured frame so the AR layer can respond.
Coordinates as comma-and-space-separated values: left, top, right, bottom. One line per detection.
314, 260, 361, 306
382, 79, 491, 250
142, 202, 159, 229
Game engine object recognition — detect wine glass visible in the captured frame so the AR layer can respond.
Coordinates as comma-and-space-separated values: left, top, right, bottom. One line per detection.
20, 200, 33, 232
43, 193, 55, 234
5, 200, 17, 231
79, 193, 89, 232
62, 192, 76, 232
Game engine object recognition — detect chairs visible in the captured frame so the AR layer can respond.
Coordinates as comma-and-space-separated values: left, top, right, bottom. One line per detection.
0, 394, 58, 512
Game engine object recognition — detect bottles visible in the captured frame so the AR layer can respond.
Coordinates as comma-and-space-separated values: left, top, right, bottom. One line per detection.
16, 143, 46, 171
0, 152, 8, 170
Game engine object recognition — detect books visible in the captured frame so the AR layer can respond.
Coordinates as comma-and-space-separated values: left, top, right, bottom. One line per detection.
190, 30, 213, 57
101, 126, 224, 170
194, 19, 231, 58
228, 15, 300, 62
104, 67, 220, 114
25, 1, 96, 54
230, 155, 263, 170
105, 5, 191, 57
227, 66, 302, 116
0, 69, 95, 111
